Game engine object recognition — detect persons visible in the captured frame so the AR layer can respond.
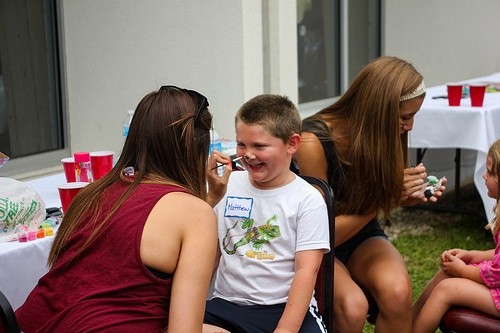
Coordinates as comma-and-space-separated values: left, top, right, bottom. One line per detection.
410, 138, 500, 333
290, 56, 448, 333
11, 84, 234, 333
201, 94, 332, 333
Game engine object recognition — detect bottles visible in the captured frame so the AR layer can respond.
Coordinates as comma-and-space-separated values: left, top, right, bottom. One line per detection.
208, 125, 223, 177
123, 109, 135, 145
74, 152, 94, 183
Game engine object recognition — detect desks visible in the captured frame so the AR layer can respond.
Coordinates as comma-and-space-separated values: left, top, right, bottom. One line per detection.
408, 72, 500, 215
0, 140, 237, 313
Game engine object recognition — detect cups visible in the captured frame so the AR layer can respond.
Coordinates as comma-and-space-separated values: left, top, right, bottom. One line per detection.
447, 83, 462, 106
469, 82, 486, 107
58, 182, 89, 215
90, 151, 115, 181
61, 157, 76, 182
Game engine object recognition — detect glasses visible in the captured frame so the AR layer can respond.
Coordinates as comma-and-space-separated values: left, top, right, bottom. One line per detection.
157, 85, 209, 129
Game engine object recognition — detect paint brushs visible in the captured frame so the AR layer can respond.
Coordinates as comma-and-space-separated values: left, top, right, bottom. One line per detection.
211, 156, 243, 170
